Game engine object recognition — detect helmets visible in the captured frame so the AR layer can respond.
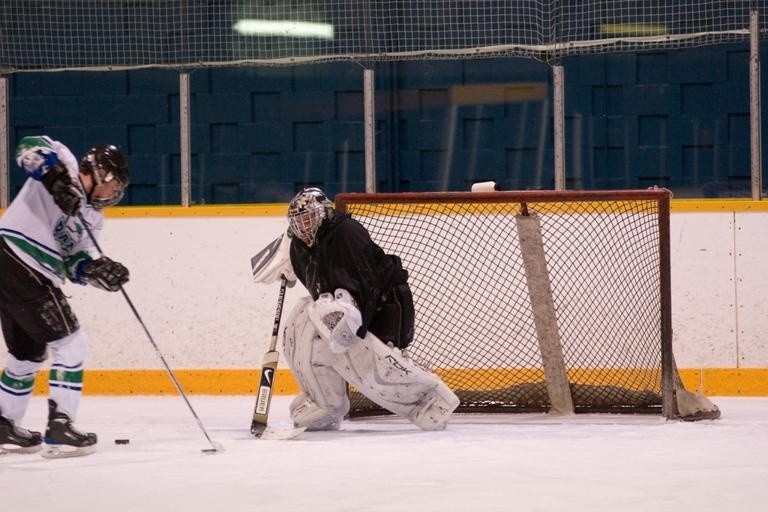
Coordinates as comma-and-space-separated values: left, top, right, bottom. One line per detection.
83, 144, 129, 209
288, 186, 333, 247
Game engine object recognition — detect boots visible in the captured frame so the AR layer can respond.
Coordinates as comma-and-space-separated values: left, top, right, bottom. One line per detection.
45, 399, 97, 447
0, 414, 42, 447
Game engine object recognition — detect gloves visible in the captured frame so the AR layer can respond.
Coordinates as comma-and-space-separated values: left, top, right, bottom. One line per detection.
81, 256, 129, 292
47, 168, 83, 215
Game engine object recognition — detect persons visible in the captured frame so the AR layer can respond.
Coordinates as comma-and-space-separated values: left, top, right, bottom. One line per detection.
0, 135, 130, 459
250, 188, 461, 431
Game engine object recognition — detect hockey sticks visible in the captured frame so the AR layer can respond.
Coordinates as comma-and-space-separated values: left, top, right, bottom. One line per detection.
78, 212, 224, 454
251, 274, 307, 440
